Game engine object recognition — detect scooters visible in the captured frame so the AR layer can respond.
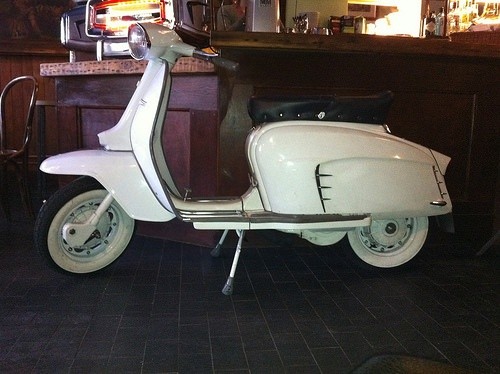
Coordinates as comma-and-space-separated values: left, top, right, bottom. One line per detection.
32, 21, 456, 296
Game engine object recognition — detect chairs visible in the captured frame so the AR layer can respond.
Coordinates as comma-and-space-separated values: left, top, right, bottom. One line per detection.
0, 75, 40, 225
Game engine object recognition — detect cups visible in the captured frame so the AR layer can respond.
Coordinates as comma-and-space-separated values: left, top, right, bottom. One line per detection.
308, 27, 329, 35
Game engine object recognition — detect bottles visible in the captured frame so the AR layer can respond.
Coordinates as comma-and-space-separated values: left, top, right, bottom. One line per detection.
422, 0, 500, 37
